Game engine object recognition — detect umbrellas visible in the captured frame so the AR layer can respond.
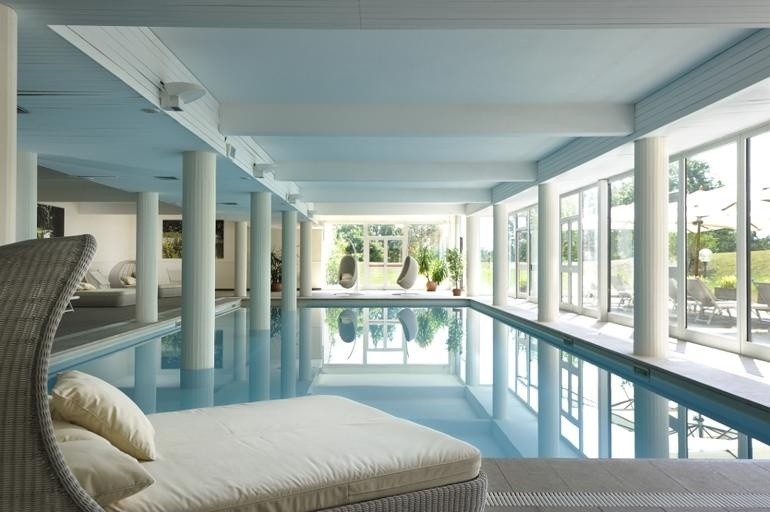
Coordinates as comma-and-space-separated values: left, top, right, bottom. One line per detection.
611, 386, 678, 440
608, 200, 672, 231
667, 405, 741, 459
668, 186, 737, 282
705, 182, 770, 235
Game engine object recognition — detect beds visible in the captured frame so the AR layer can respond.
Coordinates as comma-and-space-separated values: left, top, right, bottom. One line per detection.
72, 261, 183, 307
1, 235, 485, 510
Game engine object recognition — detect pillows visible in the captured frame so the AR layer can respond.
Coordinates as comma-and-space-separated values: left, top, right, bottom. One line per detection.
51, 368, 153, 459
50, 440, 151, 507
51, 420, 108, 446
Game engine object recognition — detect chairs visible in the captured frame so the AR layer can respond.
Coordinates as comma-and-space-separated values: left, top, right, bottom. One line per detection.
611, 272, 634, 310
668, 271, 770, 327
